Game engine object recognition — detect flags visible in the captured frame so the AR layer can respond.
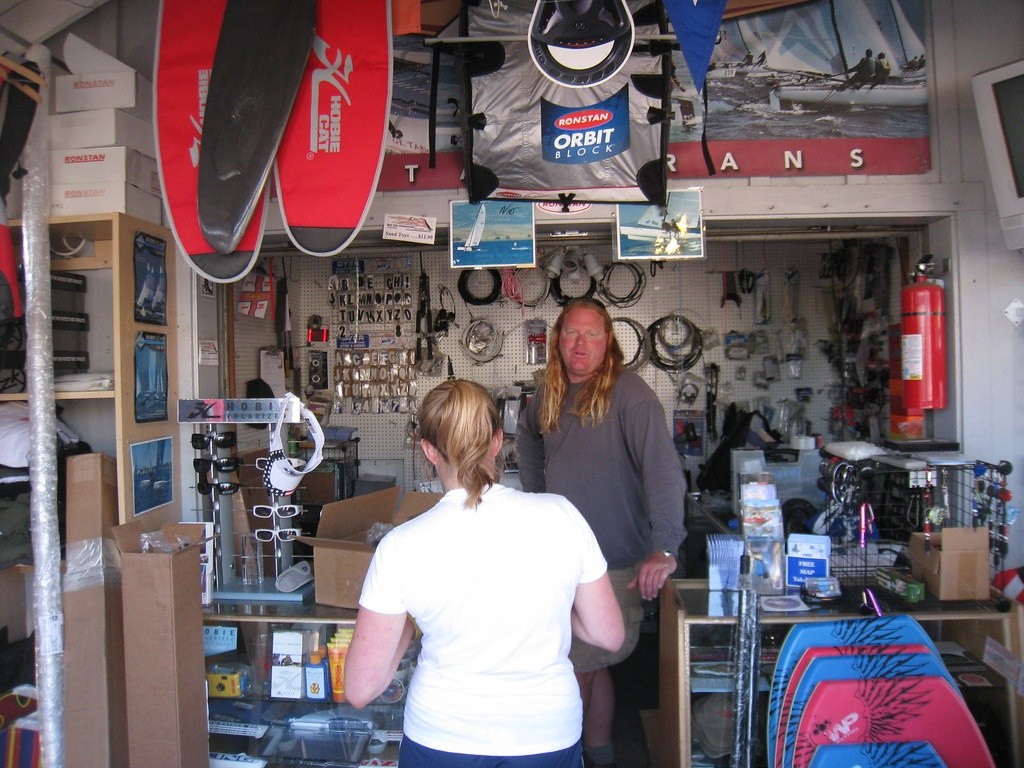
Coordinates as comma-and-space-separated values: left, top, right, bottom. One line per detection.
236, 268, 272, 319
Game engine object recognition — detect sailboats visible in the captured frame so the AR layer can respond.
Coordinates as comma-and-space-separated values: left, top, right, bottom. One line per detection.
707, 0, 928, 107
457, 204, 486, 252
620, 191, 701, 241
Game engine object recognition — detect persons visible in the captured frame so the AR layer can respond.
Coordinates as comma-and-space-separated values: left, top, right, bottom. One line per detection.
740, 50, 754, 69
753, 50, 766, 68
831, 47, 890, 92
342, 377, 627, 768
706, 63, 716, 73
902, 54, 925, 74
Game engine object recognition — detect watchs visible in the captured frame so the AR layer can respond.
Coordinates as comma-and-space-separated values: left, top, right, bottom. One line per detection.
661, 550, 673, 558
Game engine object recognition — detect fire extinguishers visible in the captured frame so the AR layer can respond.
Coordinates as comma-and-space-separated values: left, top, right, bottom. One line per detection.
901, 253, 947, 413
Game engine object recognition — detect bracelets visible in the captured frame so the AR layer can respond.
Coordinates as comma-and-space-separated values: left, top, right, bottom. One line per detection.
514, 297, 690, 767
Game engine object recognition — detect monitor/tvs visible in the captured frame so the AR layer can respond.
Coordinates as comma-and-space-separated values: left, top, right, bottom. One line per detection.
971, 58, 1024, 250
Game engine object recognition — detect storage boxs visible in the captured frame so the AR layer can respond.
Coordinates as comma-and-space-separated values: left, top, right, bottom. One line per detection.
232, 448, 275, 645
270, 629, 327, 699
292, 487, 446, 610
730, 450, 827, 514
111, 520, 208, 768
48, 33, 164, 224
62, 453, 124, 768
908, 526, 992, 602
0, 271, 90, 376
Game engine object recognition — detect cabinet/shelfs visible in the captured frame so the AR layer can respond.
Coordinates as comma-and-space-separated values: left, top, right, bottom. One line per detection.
296, 438, 362, 503
200, 600, 423, 768
661, 451, 1024, 768
1, 211, 181, 574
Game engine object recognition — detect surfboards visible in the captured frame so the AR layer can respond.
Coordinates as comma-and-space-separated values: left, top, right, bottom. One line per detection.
767, 614, 996, 768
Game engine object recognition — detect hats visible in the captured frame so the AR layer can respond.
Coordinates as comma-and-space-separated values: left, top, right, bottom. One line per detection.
263, 392, 325, 497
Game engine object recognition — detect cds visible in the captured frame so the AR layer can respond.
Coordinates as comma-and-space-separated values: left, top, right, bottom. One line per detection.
764, 598, 804, 611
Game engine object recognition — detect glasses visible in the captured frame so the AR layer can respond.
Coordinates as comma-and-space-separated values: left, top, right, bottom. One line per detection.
254, 528, 302, 542
197, 482, 251, 496
252, 504, 304, 519
193, 457, 244, 474
191, 431, 237, 450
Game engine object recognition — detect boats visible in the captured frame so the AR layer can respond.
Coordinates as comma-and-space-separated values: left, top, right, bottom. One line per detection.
511, 247, 529, 251
